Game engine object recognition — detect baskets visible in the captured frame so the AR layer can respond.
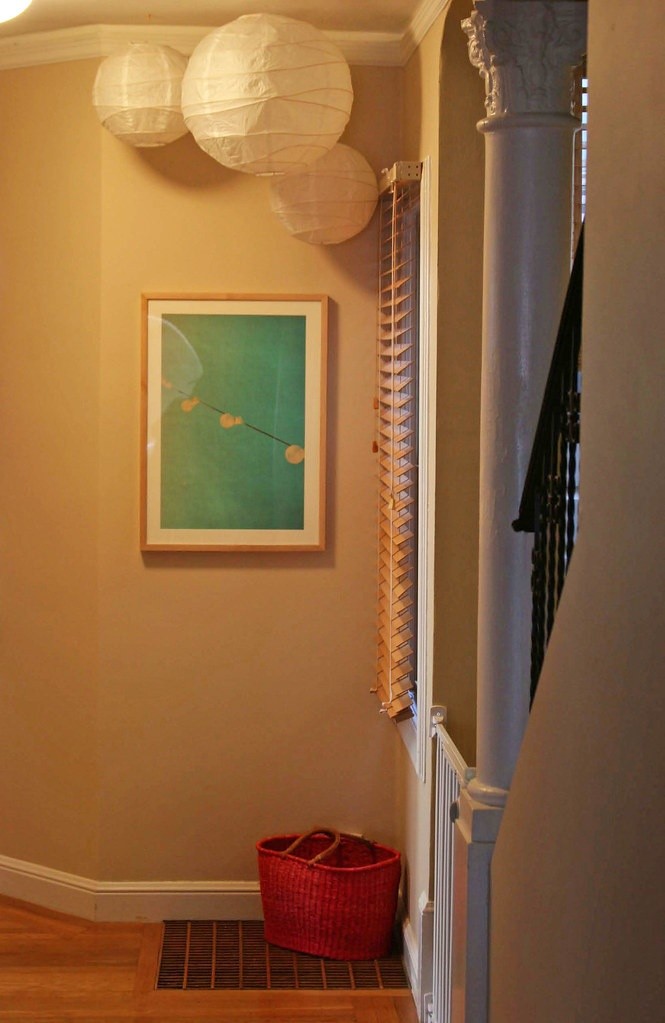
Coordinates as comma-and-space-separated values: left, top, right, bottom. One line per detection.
256, 827, 401, 961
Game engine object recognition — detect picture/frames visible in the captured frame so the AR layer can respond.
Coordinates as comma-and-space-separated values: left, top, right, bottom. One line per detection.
138, 290, 328, 552
